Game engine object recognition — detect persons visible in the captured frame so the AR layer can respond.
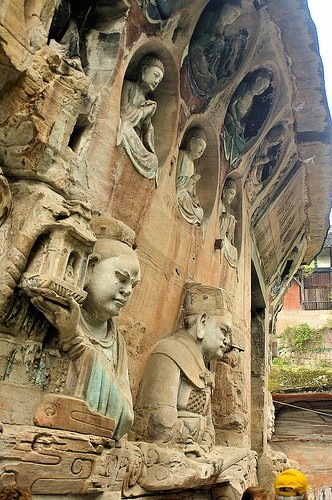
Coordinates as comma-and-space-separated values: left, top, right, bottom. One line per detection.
190, 0, 243, 102
241, 485, 276, 500
137, 280, 234, 458
273, 468, 309, 500
217, 174, 239, 269
223, 65, 274, 168
249, 122, 286, 193
28, 214, 142, 442
175, 124, 208, 226
119, 51, 166, 188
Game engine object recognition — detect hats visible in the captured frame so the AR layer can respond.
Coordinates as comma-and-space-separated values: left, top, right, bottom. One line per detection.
273, 469, 309, 495
